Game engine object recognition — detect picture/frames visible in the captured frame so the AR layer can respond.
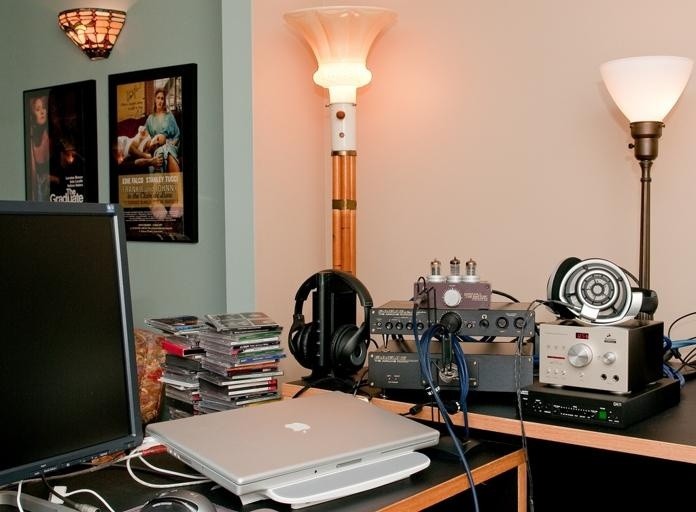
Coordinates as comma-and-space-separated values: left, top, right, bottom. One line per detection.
21, 59, 201, 246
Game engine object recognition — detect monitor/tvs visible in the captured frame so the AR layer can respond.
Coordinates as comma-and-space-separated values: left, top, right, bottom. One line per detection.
0, 199, 144, 486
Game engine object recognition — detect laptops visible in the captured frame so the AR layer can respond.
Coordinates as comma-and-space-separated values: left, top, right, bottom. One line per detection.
146, 389, 442, 496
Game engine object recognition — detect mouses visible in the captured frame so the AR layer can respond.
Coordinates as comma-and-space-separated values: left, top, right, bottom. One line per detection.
140, 489, 217, 512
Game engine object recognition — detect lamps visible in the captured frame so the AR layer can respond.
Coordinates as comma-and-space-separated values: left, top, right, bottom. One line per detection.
57, 5, 129, 60
599, 55, 695, 322
282, 2, 400, 274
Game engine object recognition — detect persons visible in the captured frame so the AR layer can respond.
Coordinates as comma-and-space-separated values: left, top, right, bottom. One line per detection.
26, 87, 183, 238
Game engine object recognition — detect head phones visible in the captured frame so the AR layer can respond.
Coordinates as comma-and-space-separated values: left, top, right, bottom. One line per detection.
287, 268, 374, 376
545, 255, 659, 326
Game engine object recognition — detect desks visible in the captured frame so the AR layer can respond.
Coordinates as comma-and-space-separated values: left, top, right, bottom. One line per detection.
279, 360, 695, 462
2, 415, 532, 511
